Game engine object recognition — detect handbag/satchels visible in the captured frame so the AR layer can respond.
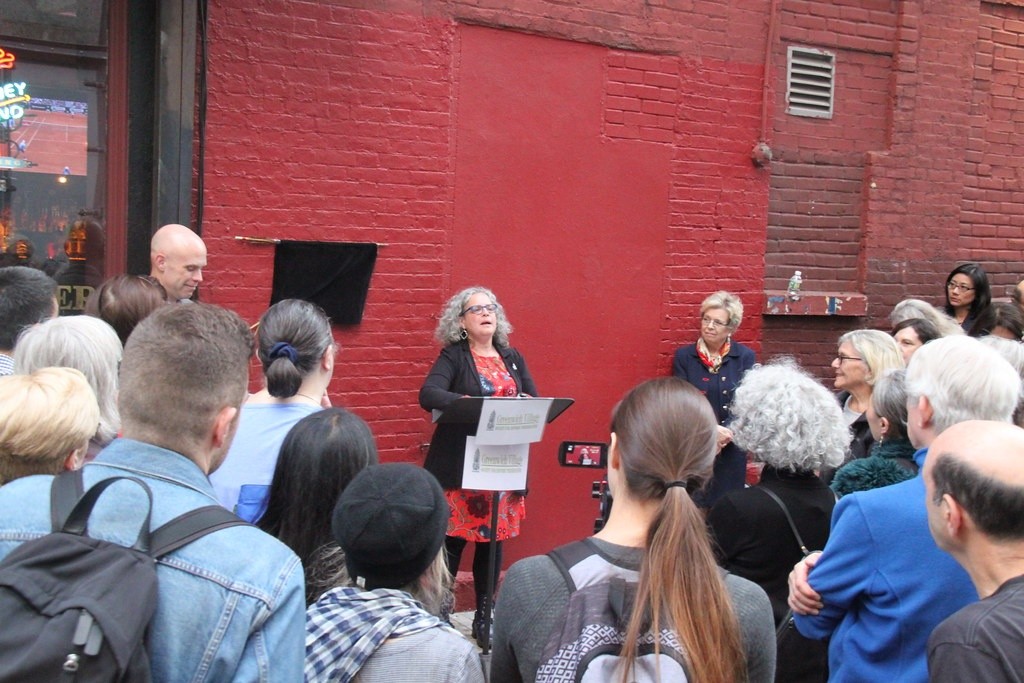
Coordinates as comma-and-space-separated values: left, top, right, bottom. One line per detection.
767, 606, 829, 683
0, 465, 258, 683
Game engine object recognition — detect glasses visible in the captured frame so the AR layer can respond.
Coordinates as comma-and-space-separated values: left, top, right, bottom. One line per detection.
460, 305, 497, 318
702, 316, 727, 329
947, 281, 975, 293
837, 354, 865, 365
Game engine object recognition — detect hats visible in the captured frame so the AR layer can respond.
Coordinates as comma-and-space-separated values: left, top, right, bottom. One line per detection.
332, 462, 447, 590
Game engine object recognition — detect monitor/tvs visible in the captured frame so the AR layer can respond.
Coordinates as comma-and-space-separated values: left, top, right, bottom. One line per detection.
0, 81, 99, 185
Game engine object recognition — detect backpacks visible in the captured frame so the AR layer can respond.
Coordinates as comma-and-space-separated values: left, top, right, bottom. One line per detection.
534, 541, 730, 683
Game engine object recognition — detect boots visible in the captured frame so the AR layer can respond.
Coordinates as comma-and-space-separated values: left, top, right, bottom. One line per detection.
471, 593, 496, 646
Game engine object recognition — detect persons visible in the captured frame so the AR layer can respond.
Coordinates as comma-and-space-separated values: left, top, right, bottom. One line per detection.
209, 300, 335, 523
418, 288, 538, 643
712, 367, 849, 683
0, 300, 306, 683
86, 274, 168, 344
489, 378, 775, 682
788, 265, 1024, 683
307, 463, 488, 683
0, 368, 100, 485
673, 292, 756, 510
251, 408, 380, 607
51, 221, 106, 286
151, 224, 207, 305
0, 266, 59, 375
14, 314, 123, 464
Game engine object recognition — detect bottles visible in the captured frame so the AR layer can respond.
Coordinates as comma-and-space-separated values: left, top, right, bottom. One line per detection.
788, 270, 804, 292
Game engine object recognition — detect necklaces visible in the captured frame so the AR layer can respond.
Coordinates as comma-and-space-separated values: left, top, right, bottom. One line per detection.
297, 393, 314, 400
